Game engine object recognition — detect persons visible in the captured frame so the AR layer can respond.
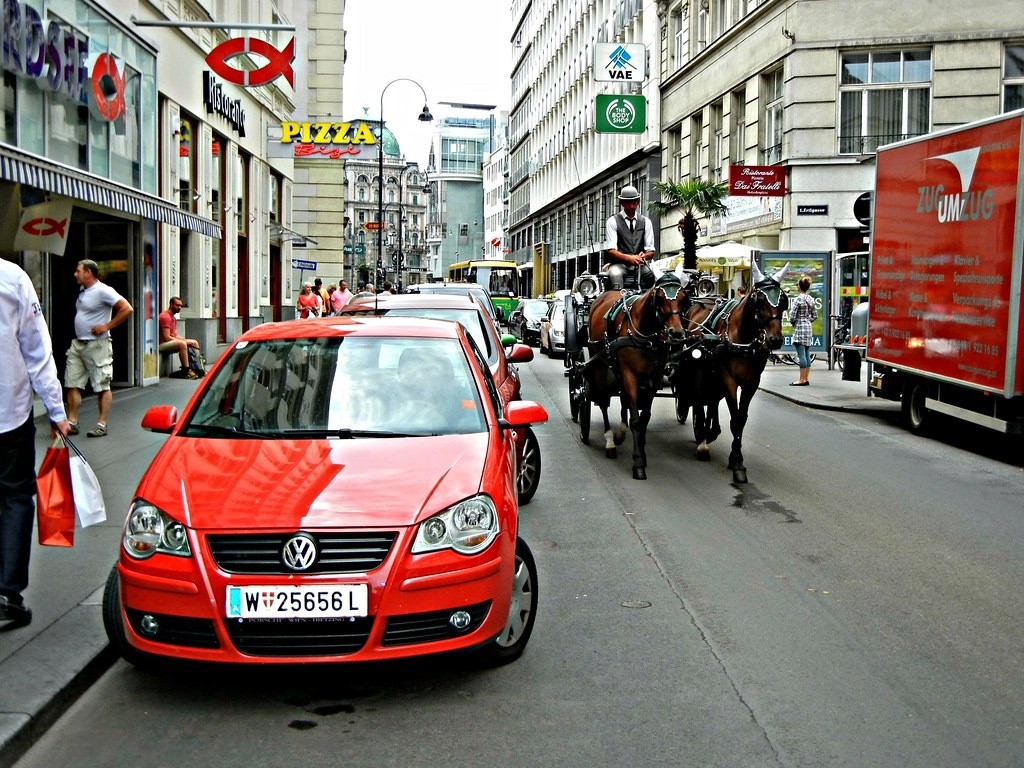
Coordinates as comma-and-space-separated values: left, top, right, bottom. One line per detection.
356, 283, 375, 295
737, 285, 745, 300
490, 272, 513, 291
297, 279, 354, 320
0, 252, 71, 635
64, 259, 134, 438
788, 276, 817, 386
358, 347, 477, 432
604, 186, 656, 291
378, 282, 393, 296
159, 297, 208, 380
464, 276, 467, 283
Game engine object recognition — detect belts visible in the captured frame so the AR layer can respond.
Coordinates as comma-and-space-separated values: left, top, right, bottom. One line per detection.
78, 339, 89, 345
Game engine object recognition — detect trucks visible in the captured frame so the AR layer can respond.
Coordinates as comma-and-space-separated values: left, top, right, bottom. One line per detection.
859, 103, 1024, 459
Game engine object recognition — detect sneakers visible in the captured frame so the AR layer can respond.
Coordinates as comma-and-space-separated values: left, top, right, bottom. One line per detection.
69, 422, 79, 434
87, 424, 107, 437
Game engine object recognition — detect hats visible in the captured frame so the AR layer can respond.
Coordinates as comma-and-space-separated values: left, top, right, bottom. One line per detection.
315, 278, 322, 285
618, 186, 640, 199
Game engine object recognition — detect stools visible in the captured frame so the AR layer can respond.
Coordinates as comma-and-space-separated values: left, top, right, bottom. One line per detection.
602, 277, 642, 289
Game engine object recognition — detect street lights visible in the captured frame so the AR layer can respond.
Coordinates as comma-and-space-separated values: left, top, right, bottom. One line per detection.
374, 77, 433, 294
396, 164, 431, 292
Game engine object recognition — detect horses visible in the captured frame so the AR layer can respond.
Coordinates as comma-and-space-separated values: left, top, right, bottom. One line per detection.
589, 258, 685, 481
687, 260, 791, 484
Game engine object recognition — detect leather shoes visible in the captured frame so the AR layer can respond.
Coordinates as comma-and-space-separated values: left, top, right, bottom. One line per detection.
0, 594, 32, 620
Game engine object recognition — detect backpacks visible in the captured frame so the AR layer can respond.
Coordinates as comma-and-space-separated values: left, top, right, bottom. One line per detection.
188, 346, 204, 378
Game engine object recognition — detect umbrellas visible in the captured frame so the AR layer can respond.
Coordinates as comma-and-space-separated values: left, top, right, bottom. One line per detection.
695, 240, 762, 300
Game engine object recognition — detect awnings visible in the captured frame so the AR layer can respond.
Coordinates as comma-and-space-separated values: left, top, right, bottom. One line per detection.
0, 141, 222, 239
270, 220, 318, 245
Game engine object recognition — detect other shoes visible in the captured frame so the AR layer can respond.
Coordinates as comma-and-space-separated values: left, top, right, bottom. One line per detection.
806, 381, 809, 385
185, 370, 198, 379
789, 382, 806, 386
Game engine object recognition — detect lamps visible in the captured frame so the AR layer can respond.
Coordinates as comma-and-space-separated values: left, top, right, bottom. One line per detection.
173, 187, 201, 200
234, 212, 257, 223
208, 201, 231, 212
265, 224, 284, 234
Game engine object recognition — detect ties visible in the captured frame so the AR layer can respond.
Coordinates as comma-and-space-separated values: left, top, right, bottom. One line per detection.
626, 217, 635, 234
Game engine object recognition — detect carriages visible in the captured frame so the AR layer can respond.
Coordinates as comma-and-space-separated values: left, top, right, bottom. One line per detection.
561, 260, 791, 486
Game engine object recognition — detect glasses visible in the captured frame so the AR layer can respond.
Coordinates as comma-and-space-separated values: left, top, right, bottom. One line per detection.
796, 284, 799, 287
77, 290, 85, 302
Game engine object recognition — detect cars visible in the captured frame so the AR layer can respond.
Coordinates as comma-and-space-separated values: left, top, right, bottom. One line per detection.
332, 276, 574, 505
103, 319, 551, 679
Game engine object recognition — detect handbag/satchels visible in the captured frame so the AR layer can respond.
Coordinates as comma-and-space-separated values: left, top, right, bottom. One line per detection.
37, 430, 75, 547
61, 433, 107, 528
307, 310, 316, 319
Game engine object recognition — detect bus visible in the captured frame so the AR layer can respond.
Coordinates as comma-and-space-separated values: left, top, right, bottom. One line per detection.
446, 259, 526, 325
